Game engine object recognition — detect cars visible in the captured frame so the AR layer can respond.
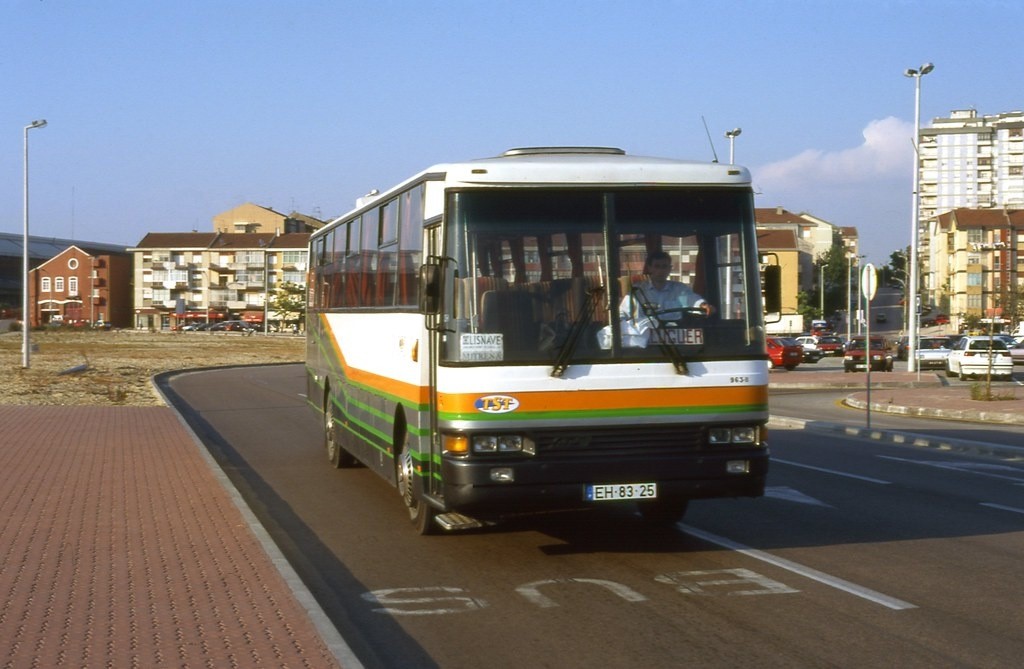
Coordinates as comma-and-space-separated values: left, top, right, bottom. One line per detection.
897, 331, 1024, 369
946, 335, 1015, 381
792, 334, 822, 360
843, 334, 894, 373
52, 315, 112, 330
876, 313, 887, 323
813, 335, 846, 358
170, 318, 295, 334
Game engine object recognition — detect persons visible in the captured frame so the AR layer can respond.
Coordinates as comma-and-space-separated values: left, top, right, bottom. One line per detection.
619, 250, 717, 324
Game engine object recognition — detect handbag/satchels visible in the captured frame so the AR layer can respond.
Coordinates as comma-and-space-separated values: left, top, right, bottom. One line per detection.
533, 310, 593, 361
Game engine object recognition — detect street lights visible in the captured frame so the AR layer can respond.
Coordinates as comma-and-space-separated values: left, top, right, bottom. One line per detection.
890, 277, 907, 331
903, 62, 935, 372
724, 127, 742, 165
820, 263, 828, 321
20, 119, 47, 370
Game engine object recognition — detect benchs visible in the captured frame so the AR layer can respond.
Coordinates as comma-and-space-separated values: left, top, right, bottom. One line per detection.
455, 275, 704, 362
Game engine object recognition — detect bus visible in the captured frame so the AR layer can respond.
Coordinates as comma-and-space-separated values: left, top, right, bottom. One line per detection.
303, 146, 781, 536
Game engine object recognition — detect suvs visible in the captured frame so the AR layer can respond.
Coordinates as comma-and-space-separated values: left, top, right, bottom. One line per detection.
763, 335, 804, 372
